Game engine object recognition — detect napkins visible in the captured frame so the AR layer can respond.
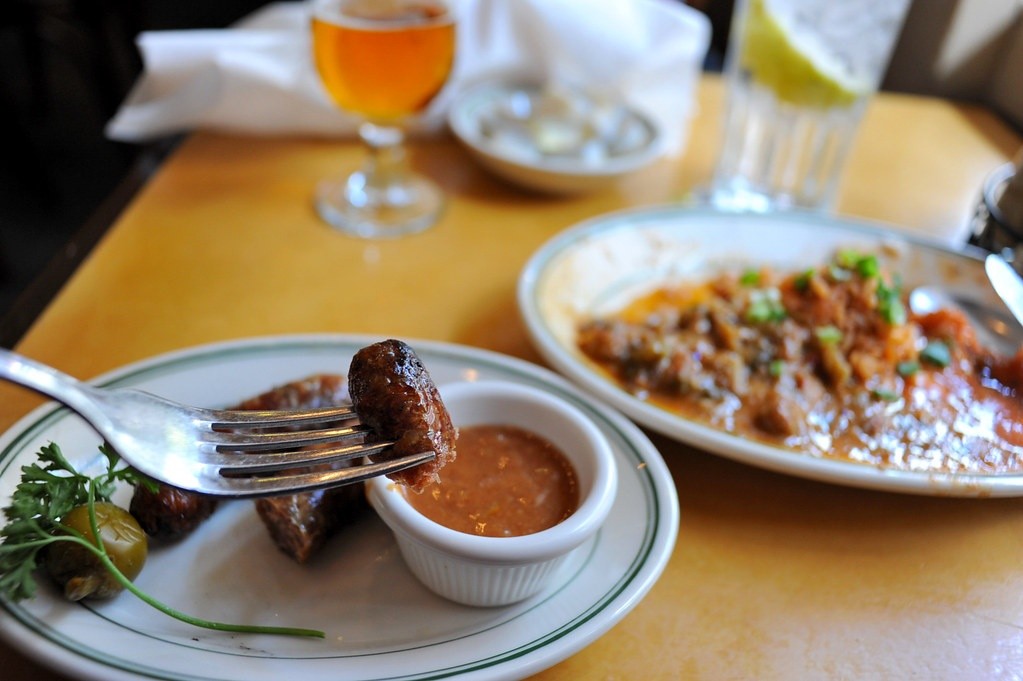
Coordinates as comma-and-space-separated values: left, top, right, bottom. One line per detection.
104, 0, 540, 132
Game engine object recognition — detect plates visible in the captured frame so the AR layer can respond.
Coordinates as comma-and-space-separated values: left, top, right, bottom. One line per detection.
0, 332, 681, 681
516, 199, 1023, 497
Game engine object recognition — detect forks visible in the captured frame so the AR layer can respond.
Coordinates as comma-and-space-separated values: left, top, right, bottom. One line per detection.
0, 345, 439, 501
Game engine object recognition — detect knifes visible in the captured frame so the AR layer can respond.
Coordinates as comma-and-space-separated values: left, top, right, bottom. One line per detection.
983, 252, 1023, 327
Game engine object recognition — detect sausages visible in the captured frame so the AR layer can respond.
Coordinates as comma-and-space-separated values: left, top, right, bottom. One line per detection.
131, 341, 462, 560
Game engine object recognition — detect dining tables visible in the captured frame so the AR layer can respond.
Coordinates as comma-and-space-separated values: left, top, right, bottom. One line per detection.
0, 73, 1023, 681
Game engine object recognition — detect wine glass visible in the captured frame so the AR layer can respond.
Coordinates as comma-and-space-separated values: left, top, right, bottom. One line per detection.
305, 0, 461, 238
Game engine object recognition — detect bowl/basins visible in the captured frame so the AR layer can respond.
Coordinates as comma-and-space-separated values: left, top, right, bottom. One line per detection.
362, 380, 619, 607
445, 62, 672, 196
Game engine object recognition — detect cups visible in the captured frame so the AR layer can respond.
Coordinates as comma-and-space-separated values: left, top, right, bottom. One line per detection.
708, 0, 914, 219
